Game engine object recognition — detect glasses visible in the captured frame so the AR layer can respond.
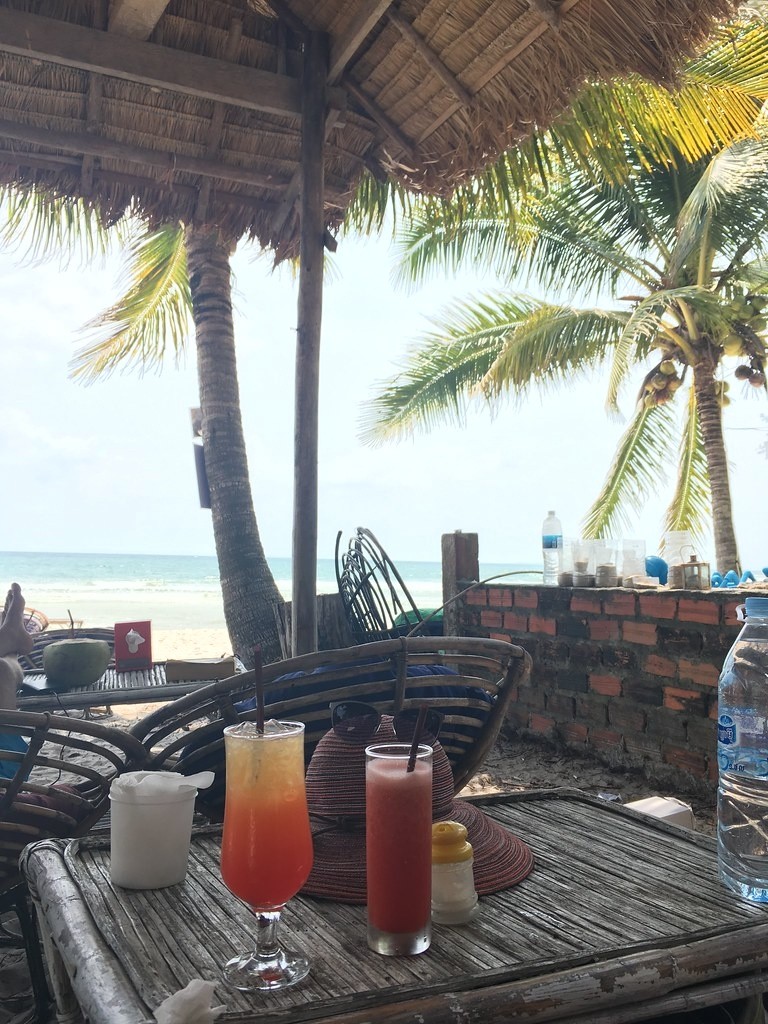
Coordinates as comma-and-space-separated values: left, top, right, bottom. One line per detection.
329, 701, 445, 748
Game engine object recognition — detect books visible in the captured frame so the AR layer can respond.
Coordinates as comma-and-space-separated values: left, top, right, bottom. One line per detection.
165, 655, 234, 680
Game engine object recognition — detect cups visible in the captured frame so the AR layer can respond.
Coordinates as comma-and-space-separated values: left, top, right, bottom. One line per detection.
365, 741, 433, 957
107, 786, 199, 890
569, 539, 594, 587
593, 538, 619, 587
664, 530, 693, 589
619, 538, 645, 589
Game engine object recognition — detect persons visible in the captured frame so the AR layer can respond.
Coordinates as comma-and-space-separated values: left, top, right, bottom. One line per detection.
0, 582, 86, 822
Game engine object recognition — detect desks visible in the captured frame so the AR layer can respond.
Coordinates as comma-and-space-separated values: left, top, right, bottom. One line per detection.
18, 785, 768, 1024
16, 654, 247, 711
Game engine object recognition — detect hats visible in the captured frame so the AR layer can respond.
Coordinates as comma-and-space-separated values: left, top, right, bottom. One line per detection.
298, 714, 534, 904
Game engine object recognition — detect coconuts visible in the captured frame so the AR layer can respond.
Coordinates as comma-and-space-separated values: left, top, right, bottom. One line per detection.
42, 637, 110, 688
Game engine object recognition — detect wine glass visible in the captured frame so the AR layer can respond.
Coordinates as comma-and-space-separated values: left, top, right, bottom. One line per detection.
221, 720, 311, 993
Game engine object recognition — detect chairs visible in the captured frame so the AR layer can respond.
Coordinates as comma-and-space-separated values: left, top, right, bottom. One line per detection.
334, 526, 444, 666
0, 709, 158, 1024
131, 634, 532, 825
16, 627, 115, 719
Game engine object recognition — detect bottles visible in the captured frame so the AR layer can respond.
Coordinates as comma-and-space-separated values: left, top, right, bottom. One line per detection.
541, 510, 563, 588
430, 820, 481, 924
715, 597, 768, 903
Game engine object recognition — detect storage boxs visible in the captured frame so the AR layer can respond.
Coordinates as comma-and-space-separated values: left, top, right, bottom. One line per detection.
623, 795, 697, 830
165, 652, 235, 681
